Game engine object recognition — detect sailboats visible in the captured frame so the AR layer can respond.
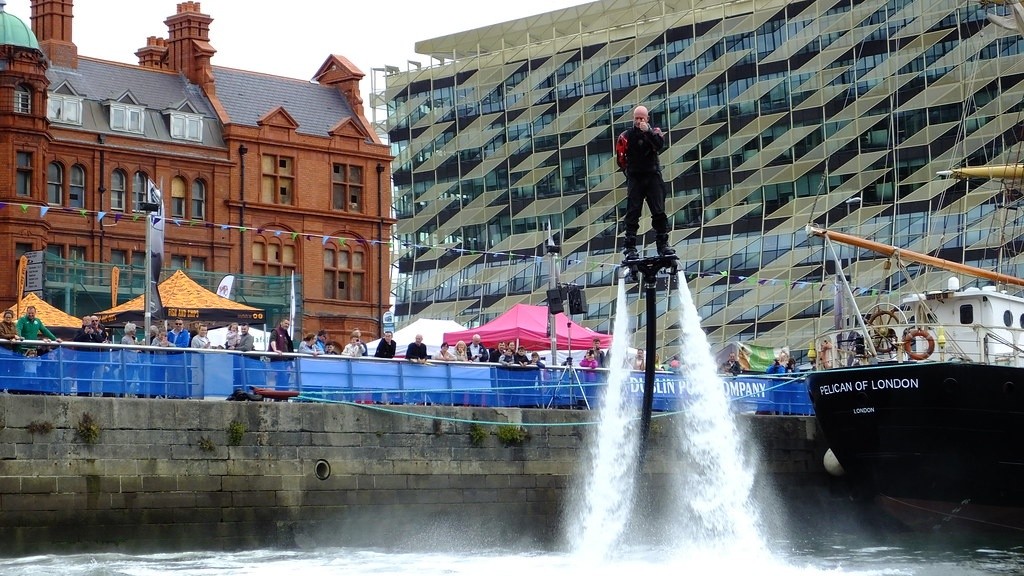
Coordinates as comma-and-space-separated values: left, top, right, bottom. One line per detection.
801, 0, 1024, 533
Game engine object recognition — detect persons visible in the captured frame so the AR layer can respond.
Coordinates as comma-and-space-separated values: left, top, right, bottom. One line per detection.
405, 334, 428, 405
617, 106, 676, 259
655, 352, 817, 415
0, 307, 368, 419
372, 330, 397, 405
431, 334, 646, 411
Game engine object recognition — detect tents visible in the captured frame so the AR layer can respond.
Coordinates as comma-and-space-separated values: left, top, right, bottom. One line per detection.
366, 302, 647, 368
0, 270, 267, 353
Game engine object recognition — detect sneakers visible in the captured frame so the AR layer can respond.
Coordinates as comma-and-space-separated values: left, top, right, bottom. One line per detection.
621, 244, 639, 263
656, 240, 675, 256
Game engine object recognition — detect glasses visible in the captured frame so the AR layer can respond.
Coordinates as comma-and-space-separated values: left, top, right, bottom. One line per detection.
176, 323, 182, 325
92, 320, 98, 322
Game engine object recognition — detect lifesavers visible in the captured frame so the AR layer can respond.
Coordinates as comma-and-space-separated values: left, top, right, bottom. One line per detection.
905, 329, 935, 359
821, 342, 832, 369
